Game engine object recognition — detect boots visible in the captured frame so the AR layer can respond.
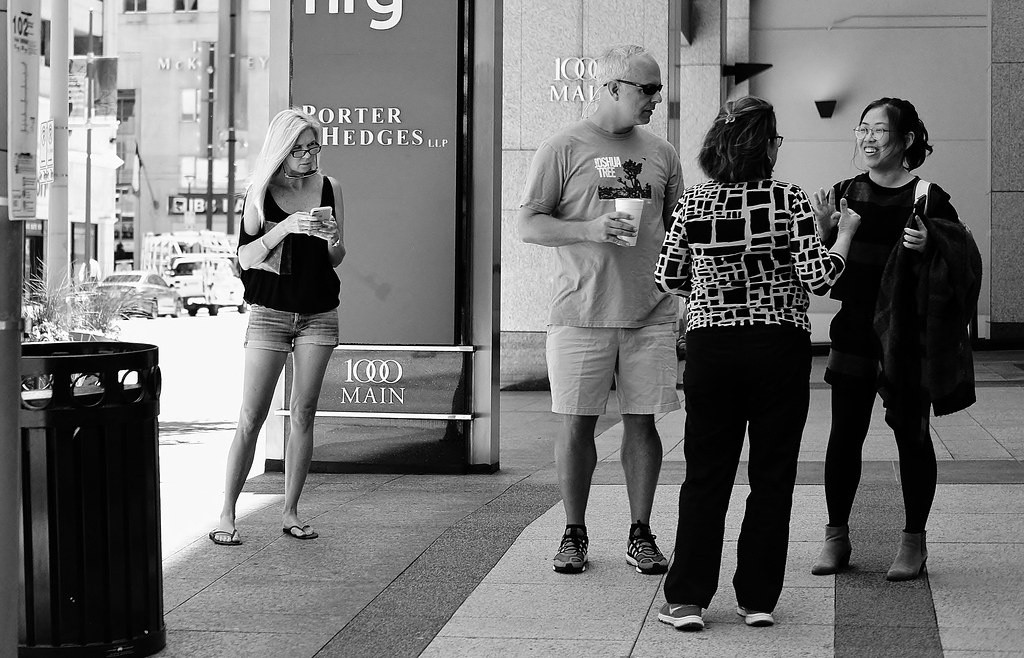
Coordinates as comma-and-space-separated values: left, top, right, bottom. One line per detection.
886, 531, 928, 581
809, 525, 851, 576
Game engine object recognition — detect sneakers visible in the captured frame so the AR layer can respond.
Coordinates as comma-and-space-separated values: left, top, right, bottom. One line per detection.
625, 521, 668, 575
658, 601, 705, 629
552, 527, 589, 574
737, 601, 775, 627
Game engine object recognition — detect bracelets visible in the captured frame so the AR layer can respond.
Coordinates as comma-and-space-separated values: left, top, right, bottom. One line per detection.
261, 237, 270, 252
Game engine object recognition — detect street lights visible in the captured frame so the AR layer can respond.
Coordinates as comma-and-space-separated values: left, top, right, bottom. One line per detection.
184, 174, 194, 230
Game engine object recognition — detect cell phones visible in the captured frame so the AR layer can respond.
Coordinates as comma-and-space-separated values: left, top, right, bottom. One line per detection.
309, 206, 332, 227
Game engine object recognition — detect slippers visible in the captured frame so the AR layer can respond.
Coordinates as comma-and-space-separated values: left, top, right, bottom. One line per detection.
209, 528, 242, 546
283, 523, 319, 540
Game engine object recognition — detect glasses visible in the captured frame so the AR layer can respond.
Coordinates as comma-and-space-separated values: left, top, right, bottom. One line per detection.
852, 126, 892, 140
770, 136, 783, 148
603, 79, 663, 95
281, 155, 319, 180
290, 141, 322, 158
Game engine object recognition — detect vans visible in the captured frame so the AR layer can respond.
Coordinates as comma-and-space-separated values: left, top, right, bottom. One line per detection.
167, 256, 247, 317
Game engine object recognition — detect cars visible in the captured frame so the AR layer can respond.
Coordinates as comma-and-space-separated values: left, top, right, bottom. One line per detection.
94, 270, 183, 319
67, 282, 97, 302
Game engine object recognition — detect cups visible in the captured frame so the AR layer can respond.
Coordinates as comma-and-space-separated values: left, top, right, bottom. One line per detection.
614, 198, 644, 246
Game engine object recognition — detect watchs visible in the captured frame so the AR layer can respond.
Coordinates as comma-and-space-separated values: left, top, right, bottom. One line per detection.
328, 234, 340, 247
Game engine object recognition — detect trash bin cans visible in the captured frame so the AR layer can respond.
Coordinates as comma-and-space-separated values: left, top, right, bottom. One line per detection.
22, 342, 166, 658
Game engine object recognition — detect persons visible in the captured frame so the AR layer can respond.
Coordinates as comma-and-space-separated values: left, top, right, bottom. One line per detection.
209, 110, 346, 546
518, 44, 686, 576
654, 97, 861, 632
813, 98, 983, 583
